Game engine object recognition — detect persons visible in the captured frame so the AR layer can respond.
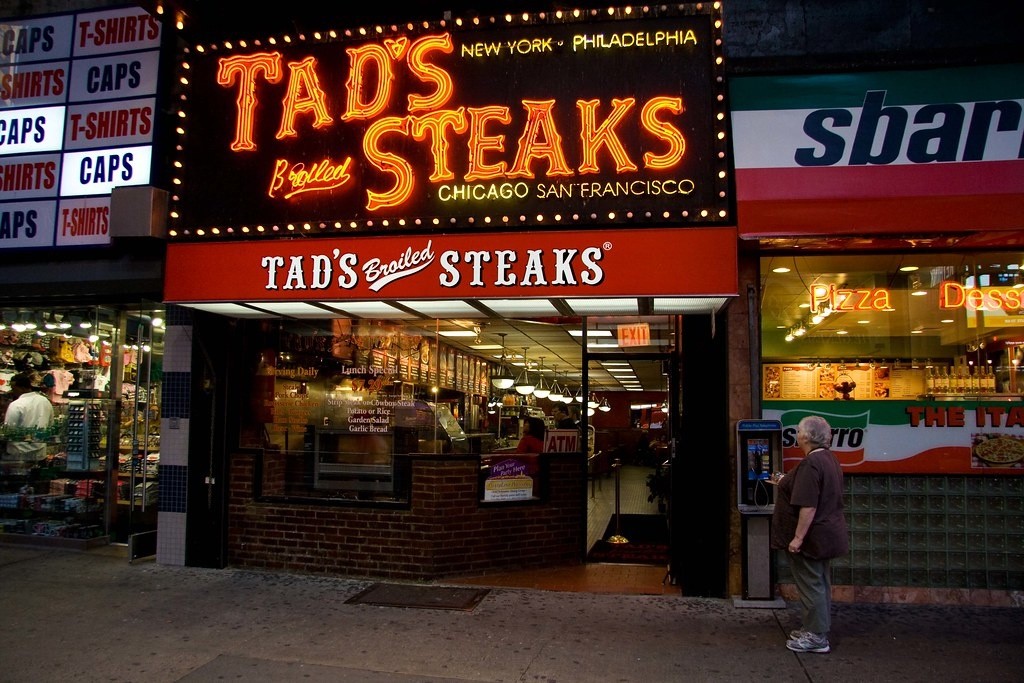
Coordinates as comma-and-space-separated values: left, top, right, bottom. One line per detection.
514, 417, 547, 478
767, 415, 849, 653
2, 373, 55, 462
550, 400, 582, 435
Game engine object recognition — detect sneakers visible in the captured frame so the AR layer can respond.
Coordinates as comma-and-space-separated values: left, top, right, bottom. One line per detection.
786, 632, 830, 653
790, 630, 829, 644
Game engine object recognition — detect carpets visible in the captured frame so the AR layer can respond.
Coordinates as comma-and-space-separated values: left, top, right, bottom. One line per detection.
587, 513, 670, 562
344, 584, 492, 612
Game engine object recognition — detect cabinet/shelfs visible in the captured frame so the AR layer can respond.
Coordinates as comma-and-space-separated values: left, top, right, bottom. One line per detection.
1, 432, 161, 550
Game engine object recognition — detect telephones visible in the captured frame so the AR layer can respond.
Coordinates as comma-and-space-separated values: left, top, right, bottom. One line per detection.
747, 437, 774, 483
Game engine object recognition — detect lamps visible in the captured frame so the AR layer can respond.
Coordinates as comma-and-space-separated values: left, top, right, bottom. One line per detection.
489, 332, 516, 390
575, 383, 583, 402
604, 402, 611, 413
513, 347, 535, 395
534, 357, 552, 398
0, 308, 100, 342
591, 392, 600, 409
588, 390, 595, 407
598, 396, 608, 411
561, 370, 573, 404
548, 364, 563, 401
784, 284, 849, 342
809, 359, 934, 370
